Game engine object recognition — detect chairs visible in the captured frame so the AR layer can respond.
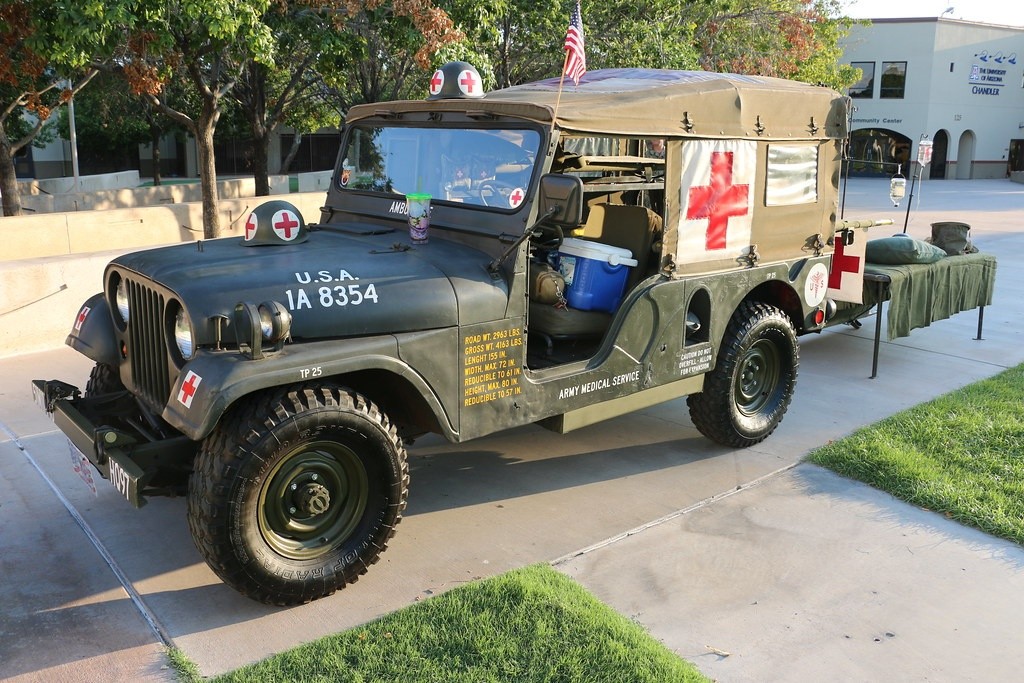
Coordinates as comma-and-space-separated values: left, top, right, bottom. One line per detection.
528, 203, 663, 357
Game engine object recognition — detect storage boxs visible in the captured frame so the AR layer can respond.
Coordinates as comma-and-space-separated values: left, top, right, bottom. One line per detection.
548, 238, 638, 312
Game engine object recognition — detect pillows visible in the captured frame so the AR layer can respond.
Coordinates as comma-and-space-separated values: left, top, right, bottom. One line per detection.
865, 236, 948, 266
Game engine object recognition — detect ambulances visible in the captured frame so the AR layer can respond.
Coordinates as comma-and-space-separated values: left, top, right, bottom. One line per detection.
32, 68, 856, 606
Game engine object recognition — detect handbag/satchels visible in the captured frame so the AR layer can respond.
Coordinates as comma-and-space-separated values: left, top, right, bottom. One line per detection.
528, 260, 566, 305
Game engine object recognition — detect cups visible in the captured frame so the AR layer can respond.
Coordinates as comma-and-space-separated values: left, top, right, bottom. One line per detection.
405, 193, 433, 243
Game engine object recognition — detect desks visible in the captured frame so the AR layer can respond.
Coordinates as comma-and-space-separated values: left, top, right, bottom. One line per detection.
861, 251, 997, 379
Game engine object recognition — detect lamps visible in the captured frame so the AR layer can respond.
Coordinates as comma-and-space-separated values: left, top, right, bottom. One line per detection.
975, 50, 1016, 64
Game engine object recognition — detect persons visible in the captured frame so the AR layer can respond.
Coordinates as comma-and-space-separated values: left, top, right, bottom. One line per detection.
644, 137, 666, 219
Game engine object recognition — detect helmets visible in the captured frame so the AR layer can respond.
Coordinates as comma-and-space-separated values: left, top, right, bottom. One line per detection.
238, 199, 311, 247
425, 61, 487, 101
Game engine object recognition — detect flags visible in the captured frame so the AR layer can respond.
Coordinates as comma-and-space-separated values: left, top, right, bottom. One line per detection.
563, 0, 587, 87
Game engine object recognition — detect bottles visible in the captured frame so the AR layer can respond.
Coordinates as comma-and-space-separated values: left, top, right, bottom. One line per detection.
890, 178, 906, 207
918, 141, 933, 167
